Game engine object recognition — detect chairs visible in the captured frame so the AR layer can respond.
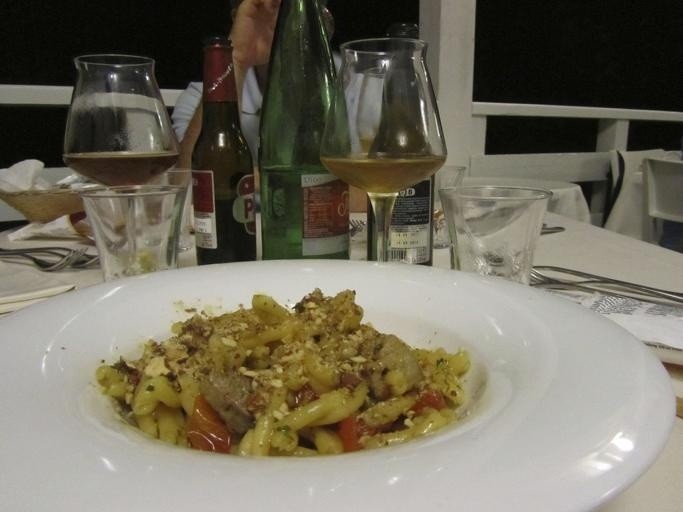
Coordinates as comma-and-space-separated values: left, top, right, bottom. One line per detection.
645, 158, 683, 251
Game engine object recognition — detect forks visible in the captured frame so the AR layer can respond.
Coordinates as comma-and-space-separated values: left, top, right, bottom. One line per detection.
528, 269, 680, 310
0, 249, 86, 273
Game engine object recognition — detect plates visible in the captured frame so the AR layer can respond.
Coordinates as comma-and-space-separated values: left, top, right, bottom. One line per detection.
0, 261, 677, 507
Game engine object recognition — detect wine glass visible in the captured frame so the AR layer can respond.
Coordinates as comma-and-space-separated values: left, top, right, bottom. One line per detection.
63, 47, 189, 261
320, 36, 448, 261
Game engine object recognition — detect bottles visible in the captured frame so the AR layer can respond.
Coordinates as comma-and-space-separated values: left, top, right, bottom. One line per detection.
259, 3, 352, 260
366, 17, 437, 261
191, 37, 258, 260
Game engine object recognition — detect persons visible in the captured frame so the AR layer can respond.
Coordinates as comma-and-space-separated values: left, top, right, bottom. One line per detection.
171, 1, 383, 212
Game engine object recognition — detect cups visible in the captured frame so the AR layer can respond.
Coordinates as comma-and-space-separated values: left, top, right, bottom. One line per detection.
78, 181, 185, 280
444, 185, 551, 283
431, 166, 463, 247
